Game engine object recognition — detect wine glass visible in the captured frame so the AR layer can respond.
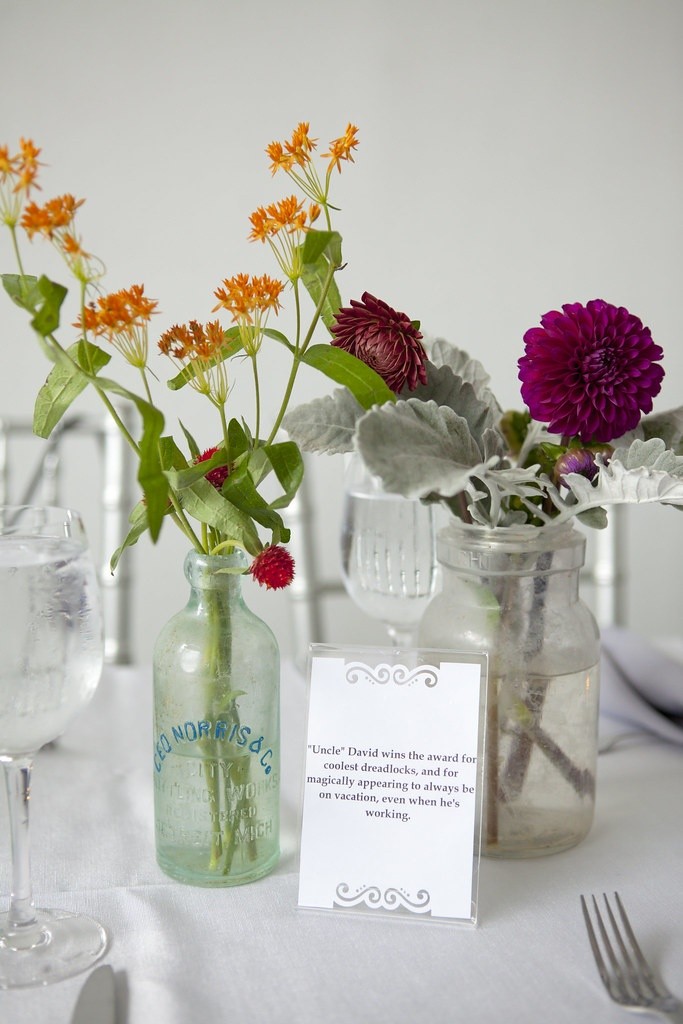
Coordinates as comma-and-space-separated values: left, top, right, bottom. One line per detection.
0, 505, 107, 990
340, 485, 448, 652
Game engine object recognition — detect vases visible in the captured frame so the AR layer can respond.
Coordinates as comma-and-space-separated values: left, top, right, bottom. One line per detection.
411, 519, 613, 865
144, 537, 287, 887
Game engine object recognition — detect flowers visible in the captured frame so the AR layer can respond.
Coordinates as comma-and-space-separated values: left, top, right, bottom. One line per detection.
0, 113, 379, 885
279, 247, 683, 867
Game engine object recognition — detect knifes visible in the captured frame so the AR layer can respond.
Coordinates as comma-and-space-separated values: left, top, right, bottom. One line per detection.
71, 964, 116, 1024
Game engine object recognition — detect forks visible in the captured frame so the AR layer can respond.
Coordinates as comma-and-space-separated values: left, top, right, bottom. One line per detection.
580, 891, 683, 1024
